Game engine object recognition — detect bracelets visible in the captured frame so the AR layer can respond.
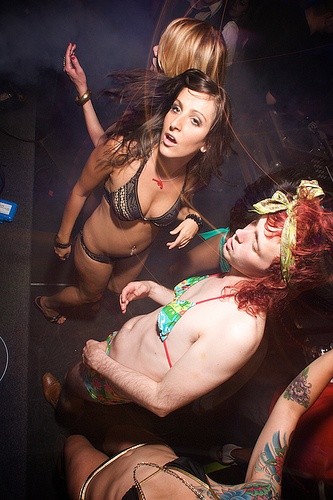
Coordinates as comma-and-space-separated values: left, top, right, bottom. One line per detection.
184, 213, 204, 230
74, 88, 92, 107
54, 235, 72, 249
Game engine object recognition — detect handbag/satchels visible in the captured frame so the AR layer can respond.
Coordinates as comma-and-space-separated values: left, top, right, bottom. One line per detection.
0, 80, 24, 114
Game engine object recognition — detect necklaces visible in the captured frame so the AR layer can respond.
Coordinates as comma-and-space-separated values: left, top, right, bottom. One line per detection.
151, 149, 187, 190
132, 461, 207, 500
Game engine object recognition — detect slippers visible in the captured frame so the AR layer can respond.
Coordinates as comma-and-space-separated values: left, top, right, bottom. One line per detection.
34, 295, 67, 324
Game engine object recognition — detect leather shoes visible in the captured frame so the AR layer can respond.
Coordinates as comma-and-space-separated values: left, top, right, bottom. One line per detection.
43, 371, 63, 408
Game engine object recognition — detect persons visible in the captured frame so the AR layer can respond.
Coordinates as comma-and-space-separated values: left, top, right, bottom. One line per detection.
214, 378, 333, 487
65, 350, 333, 500
183, 0, 250, 68
33, 68, 235, 326
42, 174, 333, 422
64, 16, 229, 150
265, 0, 333, 108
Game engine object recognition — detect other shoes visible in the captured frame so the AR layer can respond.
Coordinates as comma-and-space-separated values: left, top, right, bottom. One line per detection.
209, 444, 243, 466
